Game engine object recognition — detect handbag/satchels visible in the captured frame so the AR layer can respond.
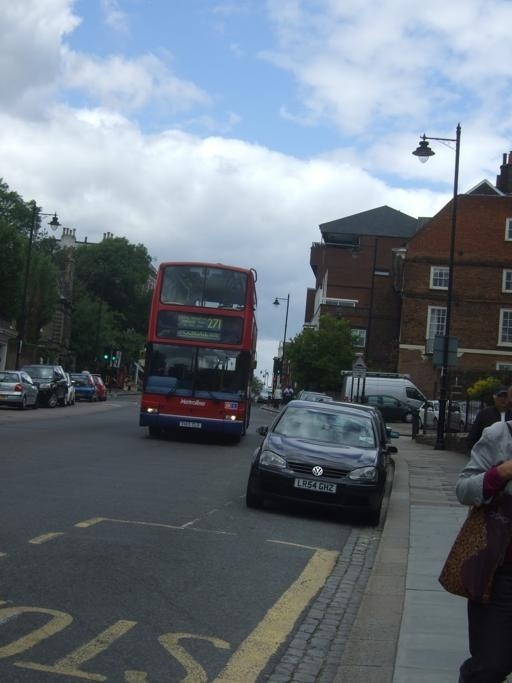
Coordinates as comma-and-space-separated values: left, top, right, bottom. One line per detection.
439, 504, 511, 603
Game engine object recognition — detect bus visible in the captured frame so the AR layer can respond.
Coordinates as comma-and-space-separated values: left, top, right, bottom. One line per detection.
138, 260, 259, 446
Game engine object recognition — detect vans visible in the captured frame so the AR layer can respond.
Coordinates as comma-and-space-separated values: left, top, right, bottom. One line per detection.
345, 375, 428, 412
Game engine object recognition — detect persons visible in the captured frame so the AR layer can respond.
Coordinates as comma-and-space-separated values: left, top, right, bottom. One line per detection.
273, 385, 282, 409
456, 420, 512, 683
283, 385, 294, 403
465, 387, 512, 456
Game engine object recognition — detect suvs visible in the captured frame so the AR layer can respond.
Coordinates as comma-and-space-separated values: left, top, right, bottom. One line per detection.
22, 364, 69, 409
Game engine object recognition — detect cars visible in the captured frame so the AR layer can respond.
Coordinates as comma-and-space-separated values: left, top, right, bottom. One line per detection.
243, 398, 399, 527
419, 398, 449, 430
354, 394, 420, 423
91, 373, 108, 401
0, 370, 40, 410
449, 400, 487, 433
70, 372, 97, 402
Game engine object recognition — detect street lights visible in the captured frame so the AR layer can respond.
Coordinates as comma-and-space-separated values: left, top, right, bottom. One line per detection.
271, 293, 290, 359
14, 201, 62, 370
411, 122, 463, 451
